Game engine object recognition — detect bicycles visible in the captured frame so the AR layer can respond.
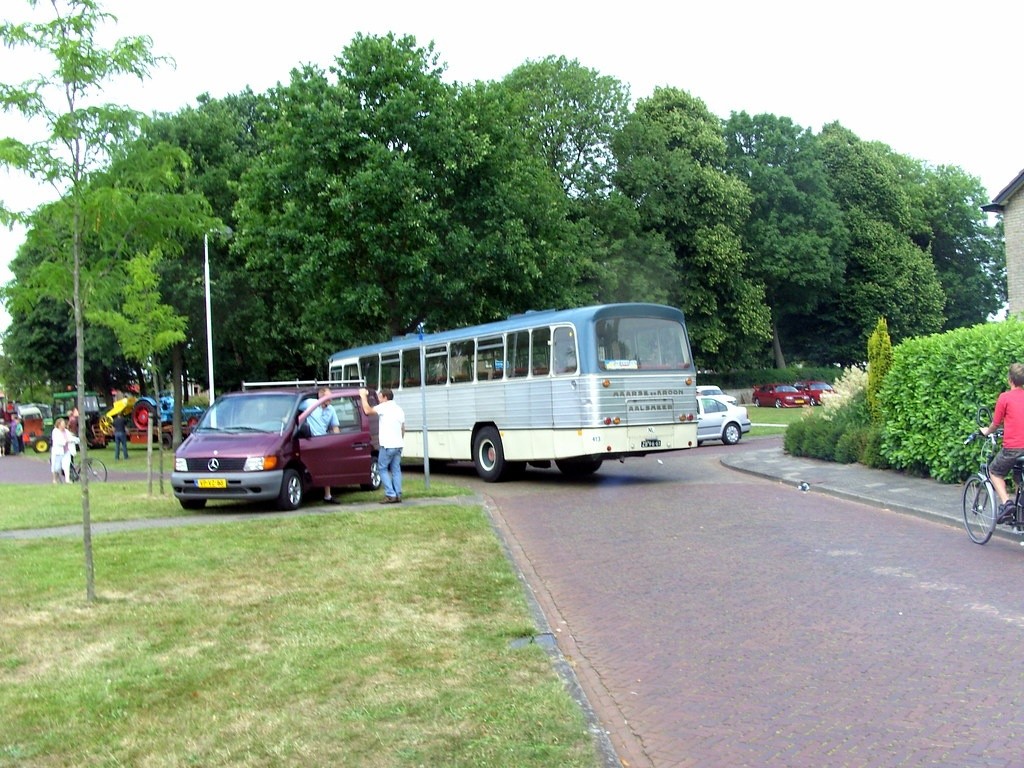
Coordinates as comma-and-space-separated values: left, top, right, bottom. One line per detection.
50, 453, 108, 484
962, 408, 1024, 545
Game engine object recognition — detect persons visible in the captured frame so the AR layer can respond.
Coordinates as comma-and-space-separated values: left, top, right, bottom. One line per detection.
11, 414, 25, 455
161, 404, 172, 426
979, 364, 1024, 525
359, 388, 405, 503
66, 407, 79, 437
52, 418, 80, 483
112, 413, 130, 459
0, 418, 10, 457
282, 387, 341, 504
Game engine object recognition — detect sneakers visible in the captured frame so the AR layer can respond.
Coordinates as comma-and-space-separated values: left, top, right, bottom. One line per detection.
996, 500, 1016, 524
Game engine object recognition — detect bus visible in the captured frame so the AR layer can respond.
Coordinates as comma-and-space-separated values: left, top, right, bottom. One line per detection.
327, 303, 698, 482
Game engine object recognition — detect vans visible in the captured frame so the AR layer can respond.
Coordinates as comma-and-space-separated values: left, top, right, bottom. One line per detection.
171, 380, 381, 509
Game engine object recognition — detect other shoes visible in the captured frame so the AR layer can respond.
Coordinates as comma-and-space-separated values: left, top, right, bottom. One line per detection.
397, 497, 401, 502
379, 496, 397, 503
324, 497, 340, 504
65, 480, 73, 484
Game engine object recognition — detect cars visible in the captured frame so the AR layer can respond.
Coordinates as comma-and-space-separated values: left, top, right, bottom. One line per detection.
5, 388, 199, 449
696, 385, 738, 405
696, 396, 750, 445
751, 383, 809, 408
794, 381, 835, 406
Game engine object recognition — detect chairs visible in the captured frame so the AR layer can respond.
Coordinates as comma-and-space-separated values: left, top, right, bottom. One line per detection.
369, 366, 549, 390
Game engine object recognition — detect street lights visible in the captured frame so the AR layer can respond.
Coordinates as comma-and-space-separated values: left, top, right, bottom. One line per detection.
203, 227, 234, 427
